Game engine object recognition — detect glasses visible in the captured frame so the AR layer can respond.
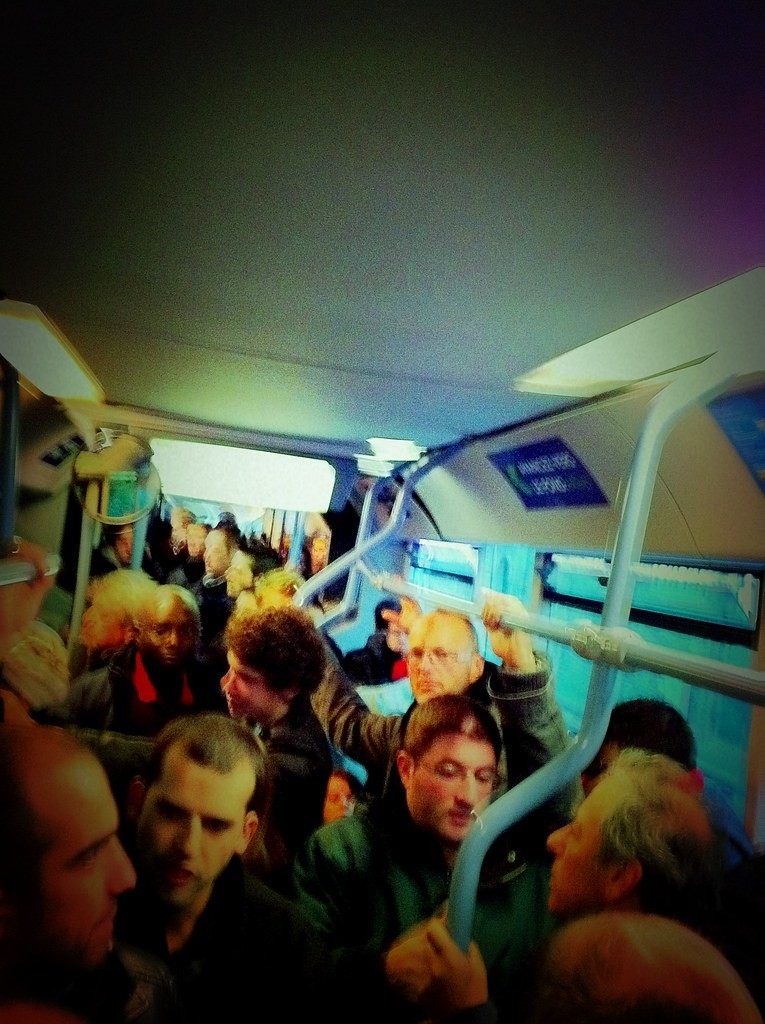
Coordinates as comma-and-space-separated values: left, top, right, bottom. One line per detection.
419, 759, 505, 792
401, 648, 459, 665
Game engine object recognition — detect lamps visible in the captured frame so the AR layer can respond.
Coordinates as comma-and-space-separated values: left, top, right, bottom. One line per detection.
0, 299, 106, 404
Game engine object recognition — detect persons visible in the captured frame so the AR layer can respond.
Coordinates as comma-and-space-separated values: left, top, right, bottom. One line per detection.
0, 509, 765, 1024
306, 591, 586, 866
